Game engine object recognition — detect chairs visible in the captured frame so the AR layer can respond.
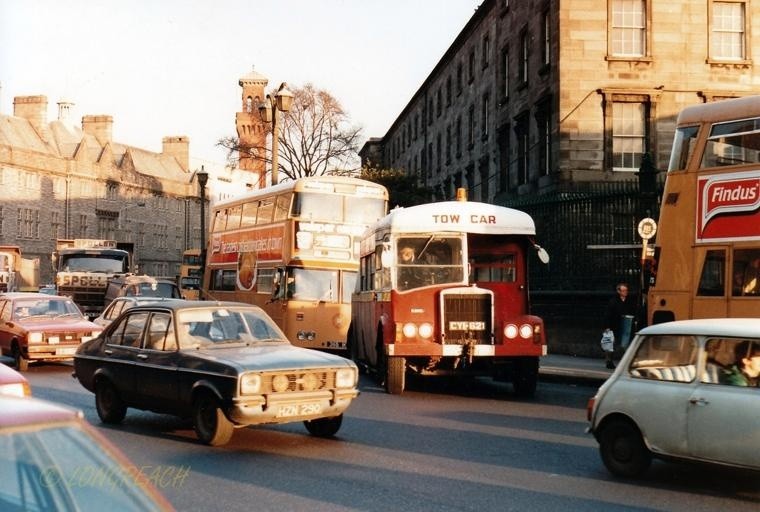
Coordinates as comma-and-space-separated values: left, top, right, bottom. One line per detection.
647, 363, 719, 384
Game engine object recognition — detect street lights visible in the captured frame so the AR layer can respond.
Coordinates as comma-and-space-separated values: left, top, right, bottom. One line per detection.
258, 81, 294, 185
196, 165, 208, 273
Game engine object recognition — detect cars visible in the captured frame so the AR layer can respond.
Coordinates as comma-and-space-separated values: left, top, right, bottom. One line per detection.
585, 318, 759, 481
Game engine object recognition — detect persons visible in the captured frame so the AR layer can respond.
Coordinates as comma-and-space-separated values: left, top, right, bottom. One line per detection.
720, 341, 760, 387
152, 321, 201, 349
600, 284, 635, 370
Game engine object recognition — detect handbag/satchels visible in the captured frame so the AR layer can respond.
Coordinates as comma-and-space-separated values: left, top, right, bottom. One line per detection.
600, 330, 615, 352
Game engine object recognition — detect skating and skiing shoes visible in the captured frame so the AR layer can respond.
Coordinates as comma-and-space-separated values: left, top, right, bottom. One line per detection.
606, 360, 615, 369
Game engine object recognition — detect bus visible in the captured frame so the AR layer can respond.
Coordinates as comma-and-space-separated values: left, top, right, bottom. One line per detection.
647, 94, 760, 326
351, 188, 549, 395
202, 176, 390, 352
178, 246, 202, 301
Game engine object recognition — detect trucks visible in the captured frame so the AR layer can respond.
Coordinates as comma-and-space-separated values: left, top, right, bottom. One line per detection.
52, 239, 134, 318
0, 246, 41, 292
